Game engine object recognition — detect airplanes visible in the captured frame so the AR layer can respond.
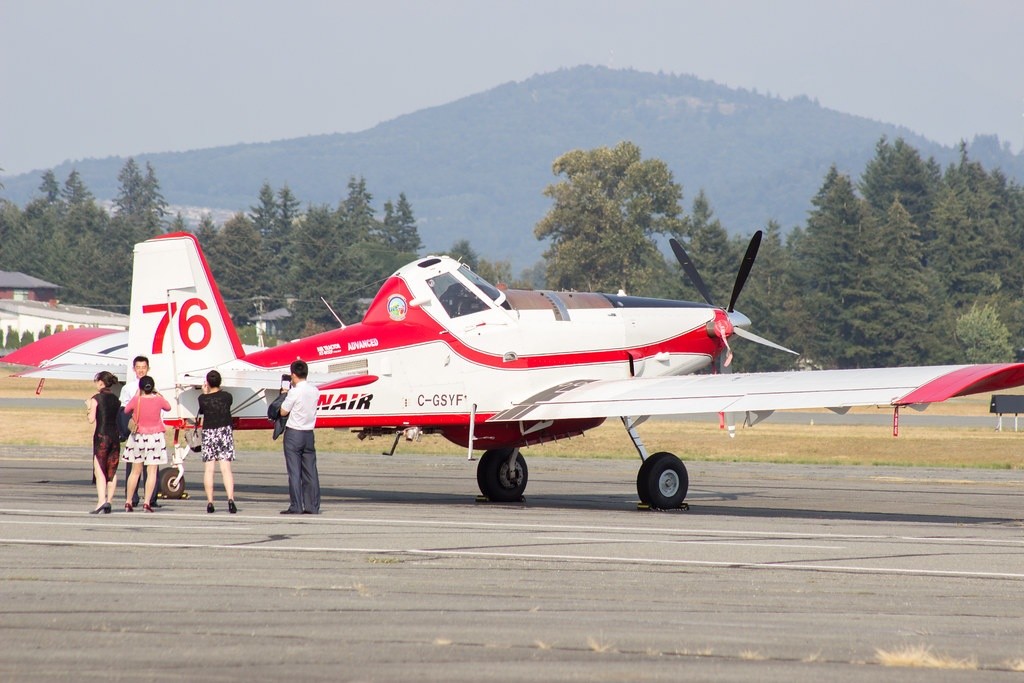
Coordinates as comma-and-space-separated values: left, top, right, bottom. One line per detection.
0, 230, 1024, 514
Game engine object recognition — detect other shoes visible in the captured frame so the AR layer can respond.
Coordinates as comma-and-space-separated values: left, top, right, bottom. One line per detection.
280, 507, 295, 514
301, 509, 312, 514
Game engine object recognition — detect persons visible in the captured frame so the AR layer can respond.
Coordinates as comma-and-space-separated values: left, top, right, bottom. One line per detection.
118, 355, 164, 508
123, 375, 171, 513
84, 371, 121, 514
280, 360, 321, 514
198, 371, 237, 514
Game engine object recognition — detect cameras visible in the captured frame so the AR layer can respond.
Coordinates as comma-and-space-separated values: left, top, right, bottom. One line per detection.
282, 375, 292, 382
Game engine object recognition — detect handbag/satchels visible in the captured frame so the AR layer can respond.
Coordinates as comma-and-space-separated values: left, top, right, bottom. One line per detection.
128, 395, 141, 435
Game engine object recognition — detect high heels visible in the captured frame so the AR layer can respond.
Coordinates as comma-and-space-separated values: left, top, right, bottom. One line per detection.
143, 503, 154, 513
228, 499, 237, 514
125, 503, 133, 513
207, 503, 214, 514
89, 502, 111, 514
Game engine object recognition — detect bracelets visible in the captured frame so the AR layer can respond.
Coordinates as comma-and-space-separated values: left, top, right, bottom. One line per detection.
87, 413, 90, 415
